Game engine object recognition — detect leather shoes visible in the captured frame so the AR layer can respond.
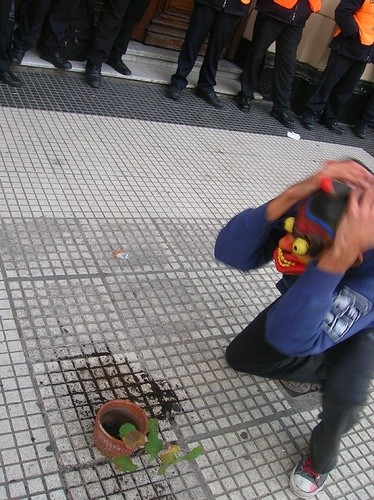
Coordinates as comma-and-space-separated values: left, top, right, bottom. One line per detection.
0, 70, 23, 87
85, 61, 101, 88
271, 109, 296, 129
40, 50, 72, 69
236, 95, 251, 112
196, 90, 224, 108
168, 84, 182, 100
104, 58, 131, 75
10, 47, 26, 66
317, 119, 345, 135
355, 121, 368, 139
302, 115, 316, 130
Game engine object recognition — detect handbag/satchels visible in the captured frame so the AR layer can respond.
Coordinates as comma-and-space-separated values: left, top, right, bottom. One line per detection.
63, 0, 95, 62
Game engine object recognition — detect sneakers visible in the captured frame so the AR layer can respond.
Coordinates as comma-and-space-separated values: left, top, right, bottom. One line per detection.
289, 448, 330, 499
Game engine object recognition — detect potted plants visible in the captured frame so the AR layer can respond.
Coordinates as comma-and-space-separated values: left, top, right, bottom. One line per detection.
96, 400, 203, 476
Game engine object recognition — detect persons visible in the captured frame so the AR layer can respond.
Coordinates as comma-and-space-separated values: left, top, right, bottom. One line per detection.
237, 0, 321, 129
302, 0, 374, 134
215, 157, 374, 500
0, 0, 22, 88
168, 0, 251, 109
86, 0, 150, 87
355, 83, 374, 139
11, 0, 80, 69
